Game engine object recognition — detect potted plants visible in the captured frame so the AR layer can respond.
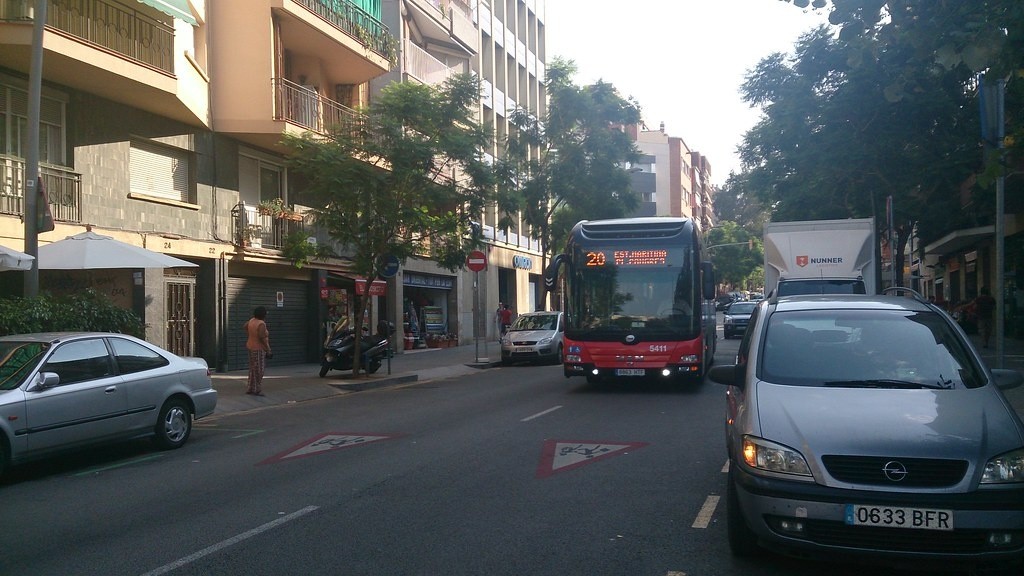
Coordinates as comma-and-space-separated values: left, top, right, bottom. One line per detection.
256, 199, 280, 215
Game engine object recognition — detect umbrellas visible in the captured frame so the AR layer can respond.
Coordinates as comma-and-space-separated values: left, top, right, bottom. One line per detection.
37, 223, 200, 270
0, 245, 35, 272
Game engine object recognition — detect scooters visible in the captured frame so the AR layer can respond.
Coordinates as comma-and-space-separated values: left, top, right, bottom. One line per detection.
319, 316, 395, 377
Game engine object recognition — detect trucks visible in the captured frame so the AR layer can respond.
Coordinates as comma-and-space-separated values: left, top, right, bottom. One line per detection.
762, 218, 884, 344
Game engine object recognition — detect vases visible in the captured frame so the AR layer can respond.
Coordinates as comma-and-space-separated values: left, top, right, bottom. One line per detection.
426, 340, 457, 349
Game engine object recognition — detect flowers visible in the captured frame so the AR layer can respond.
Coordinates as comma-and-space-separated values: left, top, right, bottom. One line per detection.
424, 332, 457, 341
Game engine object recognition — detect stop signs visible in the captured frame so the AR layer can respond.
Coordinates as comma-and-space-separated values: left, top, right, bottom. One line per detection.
466, 250, 487, 271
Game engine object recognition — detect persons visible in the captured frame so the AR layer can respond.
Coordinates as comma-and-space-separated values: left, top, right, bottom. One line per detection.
656, 287, 691, 316
493, 302, 512, 343
243, 306, 273, 396
1012, 281, 1024, 339
976, 286, 996, 348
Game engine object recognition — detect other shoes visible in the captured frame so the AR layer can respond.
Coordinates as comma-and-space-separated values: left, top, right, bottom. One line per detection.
245, 391, 255, 395
255, 391, 265, 396
498, 340, 501, 344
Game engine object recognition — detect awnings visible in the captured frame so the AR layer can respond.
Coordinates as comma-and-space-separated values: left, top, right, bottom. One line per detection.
332, 272, 387, 295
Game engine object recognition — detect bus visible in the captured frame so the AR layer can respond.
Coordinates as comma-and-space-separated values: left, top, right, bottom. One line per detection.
545, 216, 717, 392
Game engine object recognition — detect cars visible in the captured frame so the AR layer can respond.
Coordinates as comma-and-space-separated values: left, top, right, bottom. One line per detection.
0, 332, 217, 486
715, 290, 763, 311
709, 288, 1023, 576
723, 301, 760, 339
501, 311, 565, 366
